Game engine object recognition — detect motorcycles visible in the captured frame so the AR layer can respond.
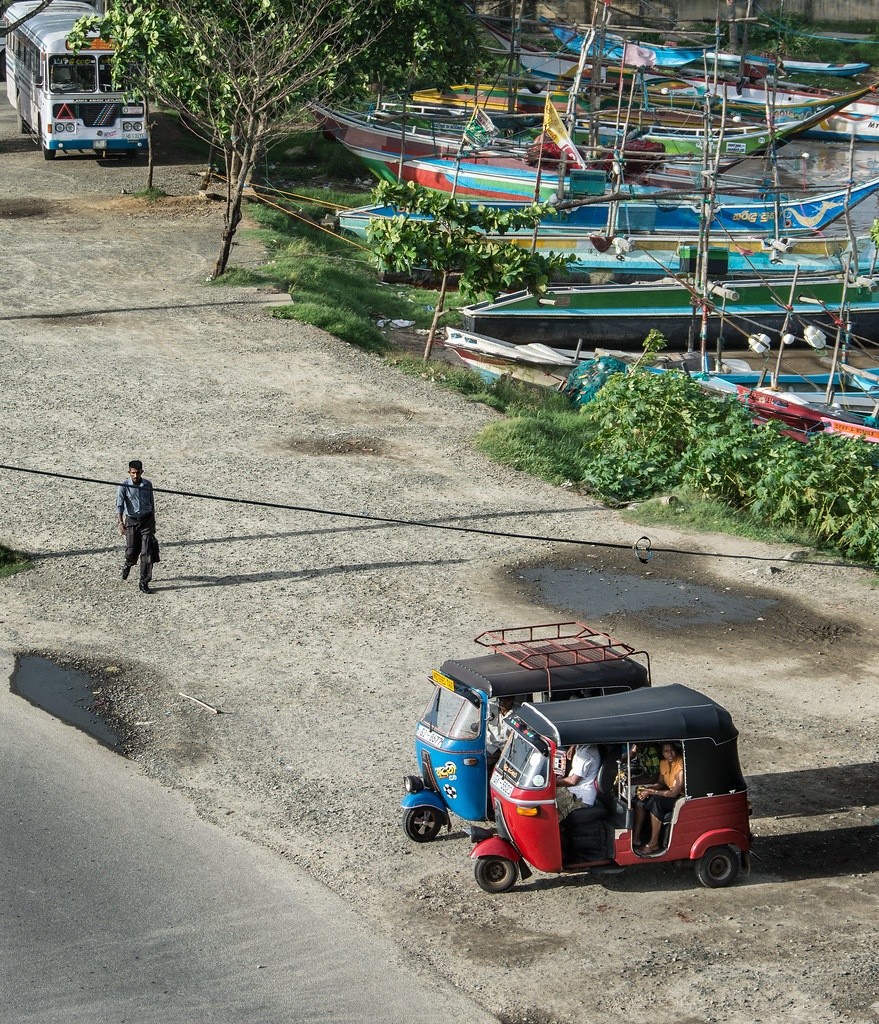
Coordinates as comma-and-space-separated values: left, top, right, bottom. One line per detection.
398, 619, 653, 842
467, 682, 761, 898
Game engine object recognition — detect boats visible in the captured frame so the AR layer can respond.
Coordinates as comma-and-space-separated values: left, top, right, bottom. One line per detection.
301, 0, 879, 460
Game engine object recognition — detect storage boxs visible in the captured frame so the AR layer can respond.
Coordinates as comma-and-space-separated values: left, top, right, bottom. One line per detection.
677, 244, 729, 276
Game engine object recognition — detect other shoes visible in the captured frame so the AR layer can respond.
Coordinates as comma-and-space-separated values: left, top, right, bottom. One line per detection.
639, 844, 660, 854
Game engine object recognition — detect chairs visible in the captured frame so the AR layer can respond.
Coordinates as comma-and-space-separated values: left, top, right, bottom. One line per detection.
568, 762, 613, 847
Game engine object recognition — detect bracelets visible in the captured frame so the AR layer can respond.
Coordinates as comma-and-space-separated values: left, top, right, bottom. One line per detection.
118, 522, 122, 525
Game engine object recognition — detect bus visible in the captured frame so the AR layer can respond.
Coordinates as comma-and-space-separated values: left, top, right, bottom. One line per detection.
3, 0, 151, 164
0, 0, 7, 83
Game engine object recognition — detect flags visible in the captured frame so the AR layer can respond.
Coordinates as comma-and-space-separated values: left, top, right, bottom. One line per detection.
543, 93, 587, 171
622, 43, 656, 66
464, 105, 501, 149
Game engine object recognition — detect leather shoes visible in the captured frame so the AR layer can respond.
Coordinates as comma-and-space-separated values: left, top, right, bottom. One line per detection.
139, 582, 151, 594
123, 564, 131, 580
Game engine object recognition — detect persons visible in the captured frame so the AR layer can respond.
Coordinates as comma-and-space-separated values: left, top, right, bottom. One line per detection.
554, 745, 603, 821
630, 742, 684, 853
116, 460, 160, 595
613, 743, 664, 796
485, 693, 521, 755
